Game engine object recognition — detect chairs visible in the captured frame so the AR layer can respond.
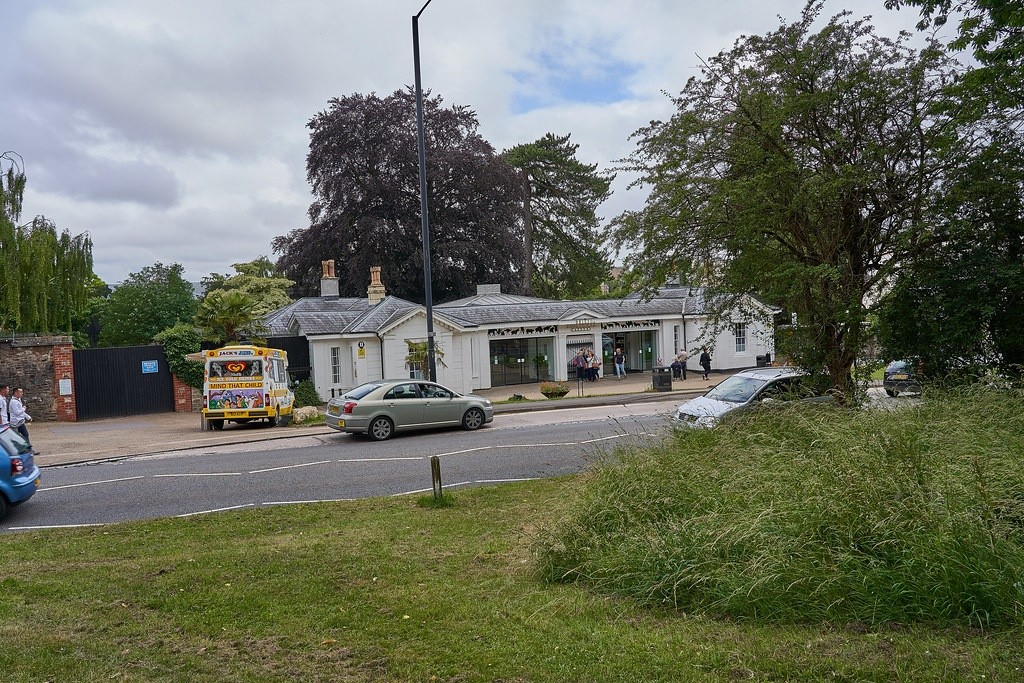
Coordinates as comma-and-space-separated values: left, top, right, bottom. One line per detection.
394, 387, 404, 398
407, 385, 416, 398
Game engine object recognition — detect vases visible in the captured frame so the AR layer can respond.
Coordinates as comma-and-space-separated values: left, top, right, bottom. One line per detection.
541, 391, 569, 398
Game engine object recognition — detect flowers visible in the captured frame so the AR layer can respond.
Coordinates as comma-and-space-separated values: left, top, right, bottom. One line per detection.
540, 380, 571, 393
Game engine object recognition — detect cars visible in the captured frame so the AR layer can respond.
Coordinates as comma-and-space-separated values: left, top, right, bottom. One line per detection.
882, 353, 981, 397
670, 364, 848, 440
323, 378, 495, 442
0, 420, 43, 525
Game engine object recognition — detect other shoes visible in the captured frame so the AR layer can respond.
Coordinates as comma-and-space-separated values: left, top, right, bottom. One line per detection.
33, 450, 41, 455
702, 374, 705, 379
705, 378, 710, 380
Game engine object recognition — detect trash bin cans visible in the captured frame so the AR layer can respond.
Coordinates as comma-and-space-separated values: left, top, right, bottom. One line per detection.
756, 355, 769, 367
651, 366, 673, 393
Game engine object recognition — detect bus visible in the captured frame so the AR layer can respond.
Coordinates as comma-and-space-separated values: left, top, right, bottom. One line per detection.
199, 344, 298, 431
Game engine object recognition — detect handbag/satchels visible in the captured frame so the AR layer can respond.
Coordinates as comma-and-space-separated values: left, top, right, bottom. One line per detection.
578, 362, 583, 368
592, 360, 598, 367
573, 357, 578, 365
585, 363, 588, 369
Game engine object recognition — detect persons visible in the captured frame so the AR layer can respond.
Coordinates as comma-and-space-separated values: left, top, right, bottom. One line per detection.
419, 385, 436, 398
614, 347, 627, 381
699, 348, 712, 380
577, 347, 601, 382
0, 383, 10, 425
9, 386, 41, 455
657, 348, 689, 380
